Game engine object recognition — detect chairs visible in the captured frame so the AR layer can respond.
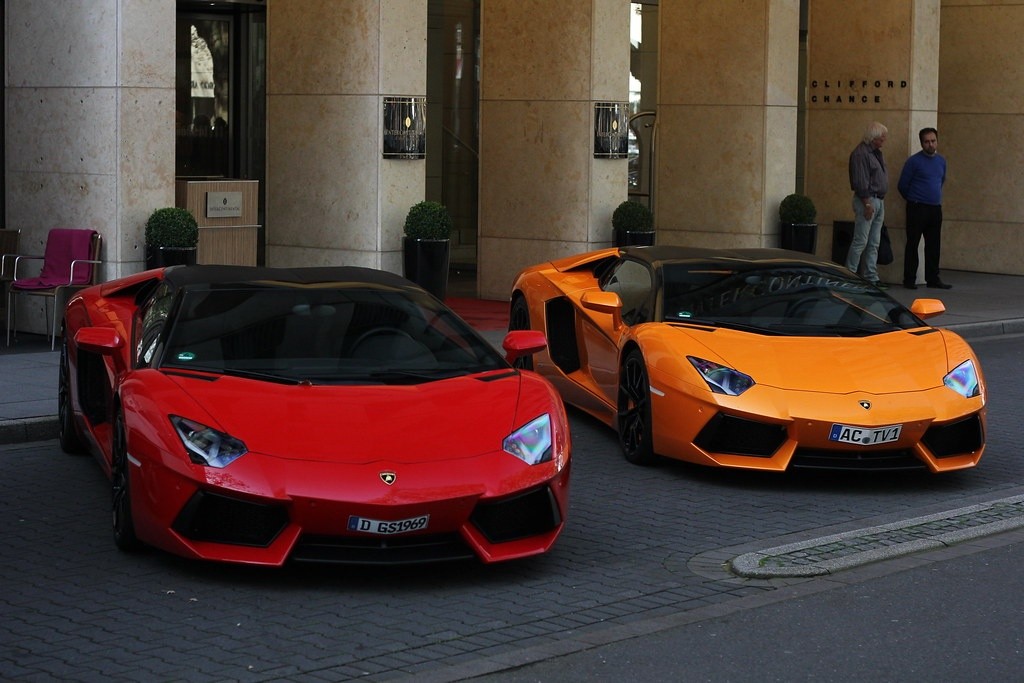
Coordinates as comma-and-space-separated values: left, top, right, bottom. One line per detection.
0, 228, 102, 350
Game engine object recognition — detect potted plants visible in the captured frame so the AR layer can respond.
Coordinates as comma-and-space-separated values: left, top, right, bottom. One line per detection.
403, 200, 453, 303
779, 194, 817, 255
611, 200, 656, 247
146, 207, 200, 271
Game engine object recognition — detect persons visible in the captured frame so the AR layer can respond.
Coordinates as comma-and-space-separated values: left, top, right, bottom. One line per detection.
896, 127, 953, 289
846, 121, 888, 288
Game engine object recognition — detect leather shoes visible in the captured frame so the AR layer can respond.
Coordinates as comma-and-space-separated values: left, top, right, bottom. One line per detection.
904, 284, 918, 289
927, 281, 953, 289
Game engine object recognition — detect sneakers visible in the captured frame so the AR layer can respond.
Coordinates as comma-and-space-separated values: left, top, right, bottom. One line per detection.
872, 281, 889, 289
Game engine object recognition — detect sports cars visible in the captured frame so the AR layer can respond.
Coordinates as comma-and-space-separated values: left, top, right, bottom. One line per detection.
509, 247, 988, 473
57, 265, 572, 564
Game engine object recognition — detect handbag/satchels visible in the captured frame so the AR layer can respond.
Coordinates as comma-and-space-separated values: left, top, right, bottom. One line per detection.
877, 224, 894, 266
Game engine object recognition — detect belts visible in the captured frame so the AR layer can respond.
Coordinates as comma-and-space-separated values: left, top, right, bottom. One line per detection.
871, 193, 886, 199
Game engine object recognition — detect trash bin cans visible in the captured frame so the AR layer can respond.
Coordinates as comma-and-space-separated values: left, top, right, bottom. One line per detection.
833, 218, 861, 275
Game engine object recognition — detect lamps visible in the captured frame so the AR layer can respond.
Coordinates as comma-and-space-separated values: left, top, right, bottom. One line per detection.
594, 102, 631, 160
382, 96, 427, 160
